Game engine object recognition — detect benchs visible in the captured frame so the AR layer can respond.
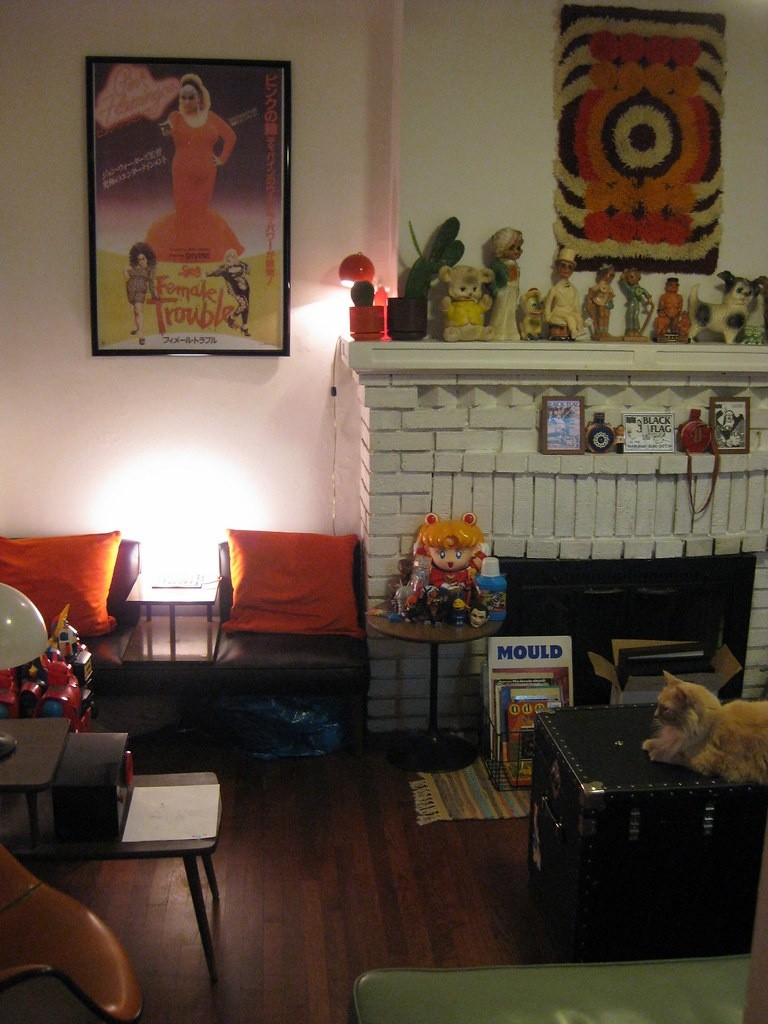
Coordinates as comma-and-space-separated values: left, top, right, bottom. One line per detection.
349, 955, 752, 1024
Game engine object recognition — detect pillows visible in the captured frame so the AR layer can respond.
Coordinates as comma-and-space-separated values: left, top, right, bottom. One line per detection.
0, 530, 122, 639
222, 530, 364, 637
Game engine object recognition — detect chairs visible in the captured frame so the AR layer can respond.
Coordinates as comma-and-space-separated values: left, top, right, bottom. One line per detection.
216, 543, 367, 757
77, 539, 143, 695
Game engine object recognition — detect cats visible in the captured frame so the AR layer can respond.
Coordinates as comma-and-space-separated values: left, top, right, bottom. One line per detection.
642, 670, 768, 786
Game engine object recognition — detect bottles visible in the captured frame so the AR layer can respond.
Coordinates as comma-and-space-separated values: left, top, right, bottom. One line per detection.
677, 408, 711, 454
585, 412, 615, 454
476, 555, 507, 620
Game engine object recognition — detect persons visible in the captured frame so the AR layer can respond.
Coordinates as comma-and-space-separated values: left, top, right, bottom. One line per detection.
654, 309, 670, 335
487, 227, 524, 340
614, 425, 626, 453
678, 310, 690, 338
413, 511, 488, 588
585, 262, 615, 337
470, 605, 490, 627
659, 278, 683, 334
544, 248, 588, 339
617, 267, 652, 336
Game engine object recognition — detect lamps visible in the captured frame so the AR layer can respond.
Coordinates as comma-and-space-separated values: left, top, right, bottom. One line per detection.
0, 582, 47, 760
338, 252, 394, 340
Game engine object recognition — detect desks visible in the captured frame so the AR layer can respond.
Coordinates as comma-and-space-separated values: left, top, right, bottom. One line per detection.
121, 572, 218, 666
366, 602, 504, 774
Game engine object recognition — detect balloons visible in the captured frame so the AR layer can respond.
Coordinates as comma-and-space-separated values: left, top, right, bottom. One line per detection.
0, 581, 48, 670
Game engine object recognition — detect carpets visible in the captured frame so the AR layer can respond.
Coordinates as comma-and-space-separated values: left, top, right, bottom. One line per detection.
409, 756, 532, 826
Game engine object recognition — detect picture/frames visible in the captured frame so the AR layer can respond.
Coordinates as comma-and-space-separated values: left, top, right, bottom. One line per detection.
85, 55, 291, 357
709, 396, 750, 455
542, 395, 585, 455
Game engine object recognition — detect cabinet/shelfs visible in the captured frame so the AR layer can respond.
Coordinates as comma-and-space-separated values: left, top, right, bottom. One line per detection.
0, 717, 221, 984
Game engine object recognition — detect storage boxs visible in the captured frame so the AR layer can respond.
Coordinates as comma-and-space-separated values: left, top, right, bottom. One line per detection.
588, 639, 742, 707
528, 703, 768, 968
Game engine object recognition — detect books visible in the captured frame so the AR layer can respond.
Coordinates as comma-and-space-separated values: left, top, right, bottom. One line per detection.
488, 635, 573, 786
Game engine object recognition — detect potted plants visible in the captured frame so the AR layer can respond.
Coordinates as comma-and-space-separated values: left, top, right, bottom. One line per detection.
350, 281, 386, 342
387, 217, 465, 341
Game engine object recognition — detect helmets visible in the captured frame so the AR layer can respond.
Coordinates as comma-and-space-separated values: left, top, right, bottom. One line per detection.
0, 580, 48, 667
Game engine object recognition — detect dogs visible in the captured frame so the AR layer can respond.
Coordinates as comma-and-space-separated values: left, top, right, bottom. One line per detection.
686, 270, 768, 344
